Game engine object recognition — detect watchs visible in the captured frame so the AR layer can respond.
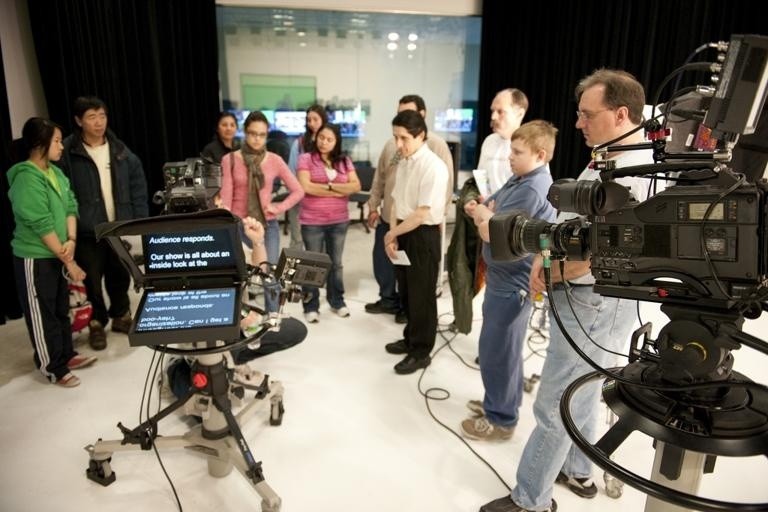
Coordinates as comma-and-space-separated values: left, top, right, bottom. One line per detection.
328, 182, 331, 192
253, 242, 264, 246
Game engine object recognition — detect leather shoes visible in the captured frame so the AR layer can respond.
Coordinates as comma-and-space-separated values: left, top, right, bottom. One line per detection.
395, 308, 409, 323
385, 339, 408, 354
394, 355, 431, 374
365, 300, 396, 315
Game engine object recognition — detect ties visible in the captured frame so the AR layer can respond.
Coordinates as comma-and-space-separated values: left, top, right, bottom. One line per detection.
385, 151, 399, 176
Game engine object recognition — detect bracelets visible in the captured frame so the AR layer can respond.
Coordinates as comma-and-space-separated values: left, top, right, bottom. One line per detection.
68, 238, 76, 242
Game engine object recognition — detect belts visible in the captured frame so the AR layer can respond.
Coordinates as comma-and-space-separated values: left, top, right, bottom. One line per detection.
553, 280, 594, 291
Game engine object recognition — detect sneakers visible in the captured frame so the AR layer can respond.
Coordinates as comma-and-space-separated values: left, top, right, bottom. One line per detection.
461, 417, 516, 442
112, 318, 134, 333
89, 329, 107, 350
55, 372, 80, 387
330, 306, 350, 317
555, 470, 598, 499
68, 355, 99, 370
304, 311, 320, 324
466, 399, 486, 415
479, 495, 558, 511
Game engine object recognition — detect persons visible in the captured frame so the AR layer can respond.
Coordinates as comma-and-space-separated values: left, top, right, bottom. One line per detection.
384, 111, 447, 374
297, 122, 360, 324
288, 104, 328, 175
156, 216, 307, 401
365, 95, 454, 324
462, 119, 558, 443
221, 111, 305, 313
6, 116, 98, 386
58, 96, 148, 351
463, 89, 529, 365
479, 71, 668, 512
203, 112, 241, 166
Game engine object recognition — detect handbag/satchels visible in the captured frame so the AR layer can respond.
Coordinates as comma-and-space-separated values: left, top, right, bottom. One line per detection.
66, 279, 92, 332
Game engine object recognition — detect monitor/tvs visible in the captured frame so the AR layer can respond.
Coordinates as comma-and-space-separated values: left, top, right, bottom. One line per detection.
327, 108, 368, 138
127, 284, 243, 346
433, 106, 475, 134
140, 223, 243, 275
275, 109, 308, 137
226, 109, 275, 139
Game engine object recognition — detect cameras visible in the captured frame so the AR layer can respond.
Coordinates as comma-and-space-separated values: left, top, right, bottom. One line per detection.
488, 33, 768, 308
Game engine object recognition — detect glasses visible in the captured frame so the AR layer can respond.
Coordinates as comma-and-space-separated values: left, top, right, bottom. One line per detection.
579, 109, 612, 118
245, 130, 267, 139
